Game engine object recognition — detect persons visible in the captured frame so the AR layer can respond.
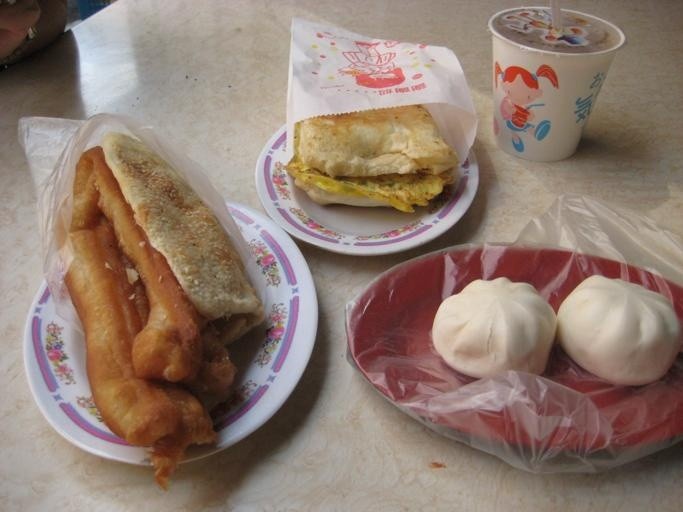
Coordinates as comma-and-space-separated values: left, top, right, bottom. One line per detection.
0, 2, 71, 71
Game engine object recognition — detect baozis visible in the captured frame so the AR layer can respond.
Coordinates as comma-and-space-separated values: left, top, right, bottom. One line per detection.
556, 275, 680, 387
431, 276, 556, 380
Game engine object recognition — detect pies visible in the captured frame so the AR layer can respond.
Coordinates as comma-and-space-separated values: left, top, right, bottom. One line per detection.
286, 104, 459, 213
100, 131, 265, 345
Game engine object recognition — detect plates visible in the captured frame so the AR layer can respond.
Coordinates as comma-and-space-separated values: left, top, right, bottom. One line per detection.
16, 197, 320, 476
258, 119, 488, 255
347, 247, 683, 473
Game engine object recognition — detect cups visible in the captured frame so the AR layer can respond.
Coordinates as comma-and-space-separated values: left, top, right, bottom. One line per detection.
486, 9, 625, 158
